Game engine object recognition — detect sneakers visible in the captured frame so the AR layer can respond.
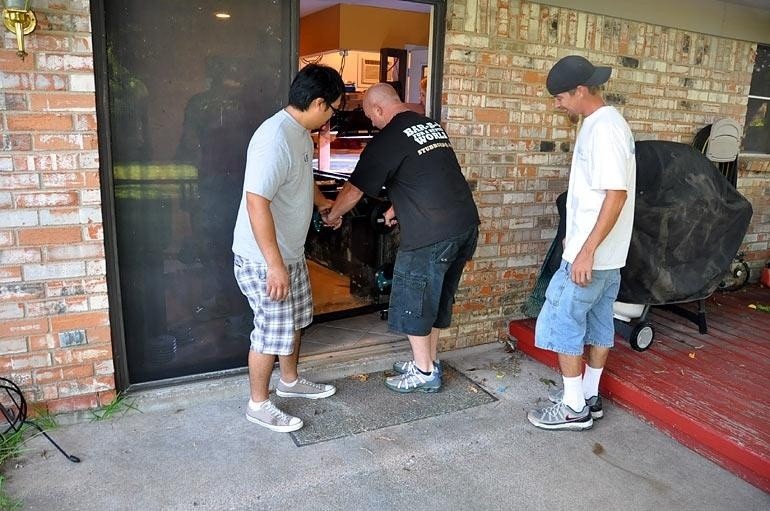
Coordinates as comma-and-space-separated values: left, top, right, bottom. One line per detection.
384, 359, 442, 393
246, 399, 303, 432
276, 378, 336, 399
528, 390, 603, 431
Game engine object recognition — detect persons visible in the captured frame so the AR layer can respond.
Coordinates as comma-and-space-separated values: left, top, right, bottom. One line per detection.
525, 55, 640, 434
320, 82, 482, 397
230, 62, 347, 435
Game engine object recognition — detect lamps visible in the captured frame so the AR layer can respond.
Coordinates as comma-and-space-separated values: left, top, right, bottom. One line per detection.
0, 0, 36, 57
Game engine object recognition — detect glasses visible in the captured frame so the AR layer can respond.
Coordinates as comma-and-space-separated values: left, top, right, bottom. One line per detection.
329, 105, 337, 116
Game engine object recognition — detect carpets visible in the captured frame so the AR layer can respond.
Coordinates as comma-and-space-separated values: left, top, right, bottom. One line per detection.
267, 361, 499, 449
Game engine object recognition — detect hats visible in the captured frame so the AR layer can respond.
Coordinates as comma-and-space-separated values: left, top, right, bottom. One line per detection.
546, 56, 612, 95
